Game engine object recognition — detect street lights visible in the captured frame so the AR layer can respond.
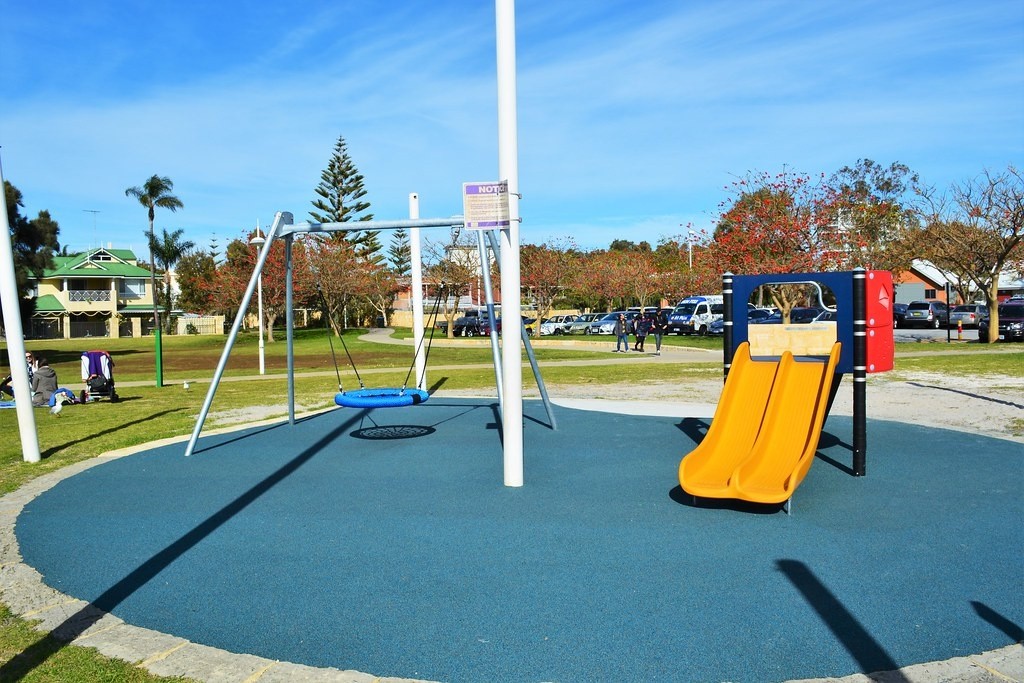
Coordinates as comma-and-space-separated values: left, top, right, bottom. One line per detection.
249, 217, 266, 375
688, 230, 694, 297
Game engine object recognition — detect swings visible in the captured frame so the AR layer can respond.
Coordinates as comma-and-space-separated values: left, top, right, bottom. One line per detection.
312, 281, 454, 409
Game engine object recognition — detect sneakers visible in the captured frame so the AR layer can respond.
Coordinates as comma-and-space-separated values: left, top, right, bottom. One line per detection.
656, 350, 660, 354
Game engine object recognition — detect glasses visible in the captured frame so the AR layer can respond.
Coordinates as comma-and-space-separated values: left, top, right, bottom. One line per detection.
26, 357, 32, 358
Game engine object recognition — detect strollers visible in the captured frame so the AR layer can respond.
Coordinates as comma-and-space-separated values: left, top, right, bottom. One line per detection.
79, 349, 119, 405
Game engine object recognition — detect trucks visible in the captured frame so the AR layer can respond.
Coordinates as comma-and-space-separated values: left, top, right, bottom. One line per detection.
435, 309, 489, 337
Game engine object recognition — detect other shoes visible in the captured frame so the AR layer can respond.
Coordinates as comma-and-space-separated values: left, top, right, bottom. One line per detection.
640, 349, 644, 352
635, 345, 638, 350
617, 351, 620, 353
625, 350, 629, 352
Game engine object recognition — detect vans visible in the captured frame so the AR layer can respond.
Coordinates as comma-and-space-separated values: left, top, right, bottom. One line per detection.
667, 295, 723, 337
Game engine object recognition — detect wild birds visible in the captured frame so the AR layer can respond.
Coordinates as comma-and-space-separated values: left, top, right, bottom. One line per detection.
49, 391, 73, 418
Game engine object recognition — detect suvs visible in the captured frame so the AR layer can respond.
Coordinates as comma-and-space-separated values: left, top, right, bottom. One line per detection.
904, 300, 955, 329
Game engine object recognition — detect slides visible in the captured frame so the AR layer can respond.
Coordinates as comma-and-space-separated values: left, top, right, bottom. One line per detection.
676, 342, 842, 504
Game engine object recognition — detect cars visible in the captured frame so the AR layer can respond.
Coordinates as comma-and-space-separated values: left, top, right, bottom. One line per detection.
948, 304, 991, 329
892, 303, 909, 329
569, 313, 608, 335
589, 311, 672, 335
522, 318, 552, 336
534, 315, 585, 336
706, 305, 837, 339
978, 294, 1024, 342
486, 315, 529, 336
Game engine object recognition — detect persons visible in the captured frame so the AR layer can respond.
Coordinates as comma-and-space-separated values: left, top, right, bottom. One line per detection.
632, 309, 652, 352
614, 314, 631, 353
651, 307, 668, 354
24, 350, 39, 387
30, 357, 58, 404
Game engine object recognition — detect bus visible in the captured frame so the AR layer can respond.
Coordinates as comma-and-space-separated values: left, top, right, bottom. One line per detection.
628, 307, 676, 313
